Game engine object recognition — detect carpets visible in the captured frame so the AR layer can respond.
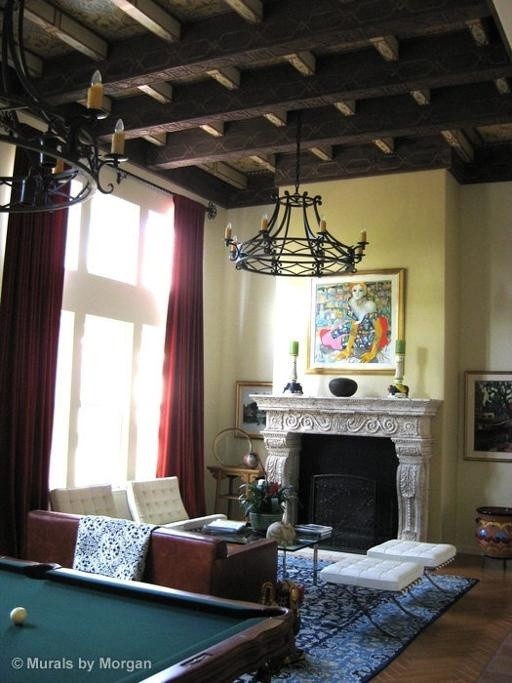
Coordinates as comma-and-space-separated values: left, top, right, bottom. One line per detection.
232, 550, 479, 683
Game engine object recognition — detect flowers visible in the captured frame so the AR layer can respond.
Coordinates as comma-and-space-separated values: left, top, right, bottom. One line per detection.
237, 474, 304, 514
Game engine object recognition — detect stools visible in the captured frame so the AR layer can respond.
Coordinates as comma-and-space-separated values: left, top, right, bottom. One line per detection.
365, 538, 456, 608
322, 554, 425, 639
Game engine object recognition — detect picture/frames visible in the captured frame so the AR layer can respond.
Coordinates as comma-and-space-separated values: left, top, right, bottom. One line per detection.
233, 380, 273, 439
303, 265, 406, 376
463, 369, 511, 462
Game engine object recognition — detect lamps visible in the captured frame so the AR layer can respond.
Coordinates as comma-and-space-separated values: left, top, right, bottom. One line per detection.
0, 0, 129, 214
395, 338, 405, 352
290, 340, 299, 354
223, 106, 371, 278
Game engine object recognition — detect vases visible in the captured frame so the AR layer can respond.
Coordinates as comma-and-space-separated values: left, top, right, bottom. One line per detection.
249, 510, 283, 532
475, 505, 512, 557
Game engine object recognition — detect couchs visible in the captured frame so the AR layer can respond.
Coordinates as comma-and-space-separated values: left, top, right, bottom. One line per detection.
24, 510, 278, 604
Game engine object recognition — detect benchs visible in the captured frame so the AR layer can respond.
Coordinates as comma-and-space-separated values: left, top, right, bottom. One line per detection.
127, 475, 228, 534
49, 484, 120, 519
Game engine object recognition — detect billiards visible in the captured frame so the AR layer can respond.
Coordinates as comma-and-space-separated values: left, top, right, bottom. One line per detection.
11, 608, 27, 624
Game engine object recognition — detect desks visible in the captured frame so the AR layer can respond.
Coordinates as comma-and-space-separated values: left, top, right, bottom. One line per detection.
1, 555, 294, 682
208, 463, 265, 520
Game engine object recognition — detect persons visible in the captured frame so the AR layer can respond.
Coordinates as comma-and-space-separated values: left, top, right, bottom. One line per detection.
322, 283, 383, 364
